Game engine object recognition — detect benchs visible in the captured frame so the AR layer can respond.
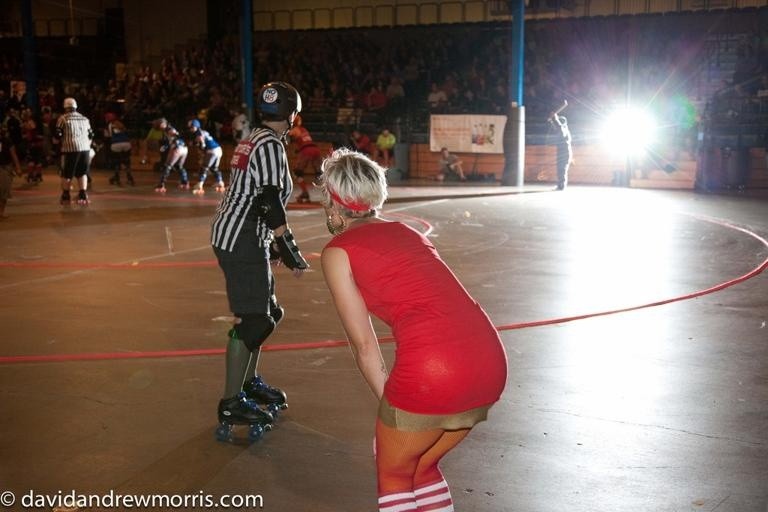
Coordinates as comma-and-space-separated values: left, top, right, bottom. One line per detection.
295, 80, 553, 146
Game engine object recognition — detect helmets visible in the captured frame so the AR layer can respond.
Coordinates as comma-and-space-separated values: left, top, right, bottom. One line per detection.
256, 80, 303, 118
188, 119, 201, 128
63, 97, 78, 109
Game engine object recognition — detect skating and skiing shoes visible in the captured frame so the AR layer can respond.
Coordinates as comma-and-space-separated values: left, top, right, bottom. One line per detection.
215, 376, 288, 442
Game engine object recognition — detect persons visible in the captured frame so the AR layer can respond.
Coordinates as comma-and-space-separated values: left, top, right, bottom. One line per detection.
310, 147, 508, 512
208, 80, 310, 442
1, 16, 767, 209
